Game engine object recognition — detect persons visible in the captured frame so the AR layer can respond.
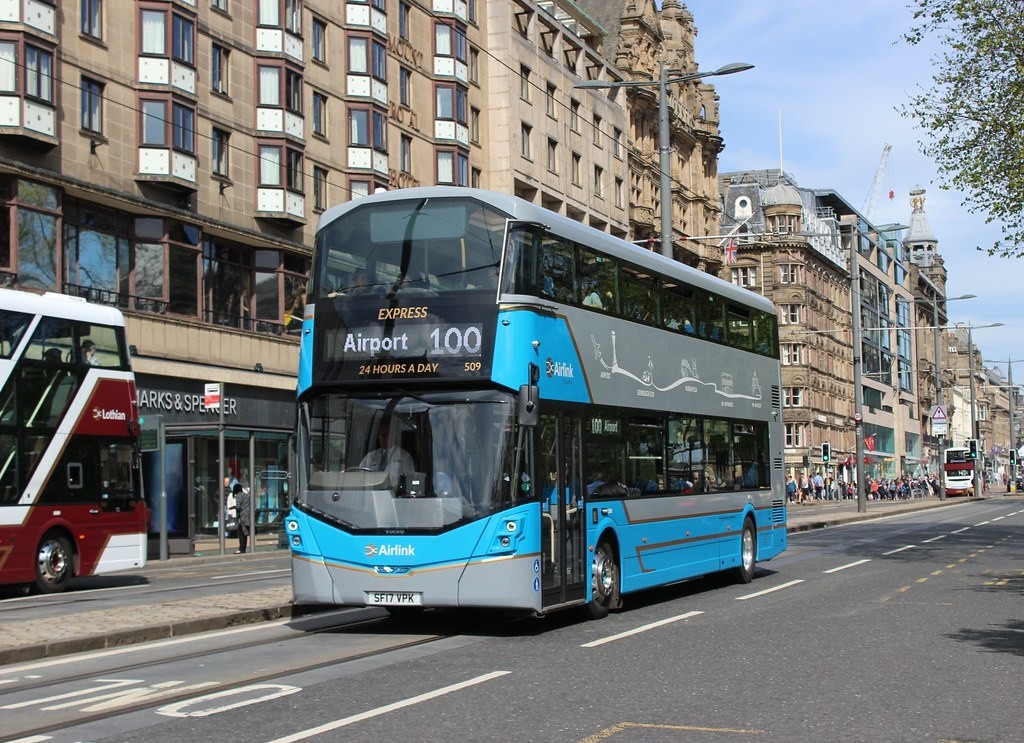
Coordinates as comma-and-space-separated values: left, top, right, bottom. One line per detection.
785, 469, 940, 506
232, 484, 250, 554
359, 424, 415, 489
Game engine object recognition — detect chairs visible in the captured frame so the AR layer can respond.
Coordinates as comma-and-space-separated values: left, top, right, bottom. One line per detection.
43, 348, 72, 365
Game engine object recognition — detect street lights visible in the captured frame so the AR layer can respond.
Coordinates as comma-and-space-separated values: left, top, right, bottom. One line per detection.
982, 356, 1024, 494
792, 224, 912, 514
573, 61, 754, 260
968, 319, 1004, 499
887, 291, 976, 501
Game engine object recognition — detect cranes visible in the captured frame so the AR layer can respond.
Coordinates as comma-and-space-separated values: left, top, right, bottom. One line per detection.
860, 141, 895, 219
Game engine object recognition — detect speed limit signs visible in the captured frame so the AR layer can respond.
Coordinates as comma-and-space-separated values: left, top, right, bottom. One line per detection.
854, 412, 863, 421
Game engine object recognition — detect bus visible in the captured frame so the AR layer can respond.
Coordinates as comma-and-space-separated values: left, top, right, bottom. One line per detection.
0, 286, 148, 593
944, 448, 984, 497
280, 187, 789, 619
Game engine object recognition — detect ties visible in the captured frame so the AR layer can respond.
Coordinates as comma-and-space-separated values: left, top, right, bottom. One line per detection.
377, 452, 389, 471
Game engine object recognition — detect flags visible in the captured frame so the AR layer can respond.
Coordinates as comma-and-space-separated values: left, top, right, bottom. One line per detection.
724, 238, 738, 265
864, 434, 875, 452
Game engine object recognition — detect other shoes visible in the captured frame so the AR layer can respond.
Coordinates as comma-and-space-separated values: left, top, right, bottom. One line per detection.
233, 551, 241, 554
225, 531, 229, 537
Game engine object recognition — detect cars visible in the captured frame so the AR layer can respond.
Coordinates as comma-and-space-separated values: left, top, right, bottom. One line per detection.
1007, 477, 1024, 492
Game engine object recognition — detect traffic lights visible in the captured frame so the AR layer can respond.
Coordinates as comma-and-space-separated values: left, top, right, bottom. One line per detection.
1009, 449, 1016, 465
821, 443, 831, 462
968, 440, 978, 459
